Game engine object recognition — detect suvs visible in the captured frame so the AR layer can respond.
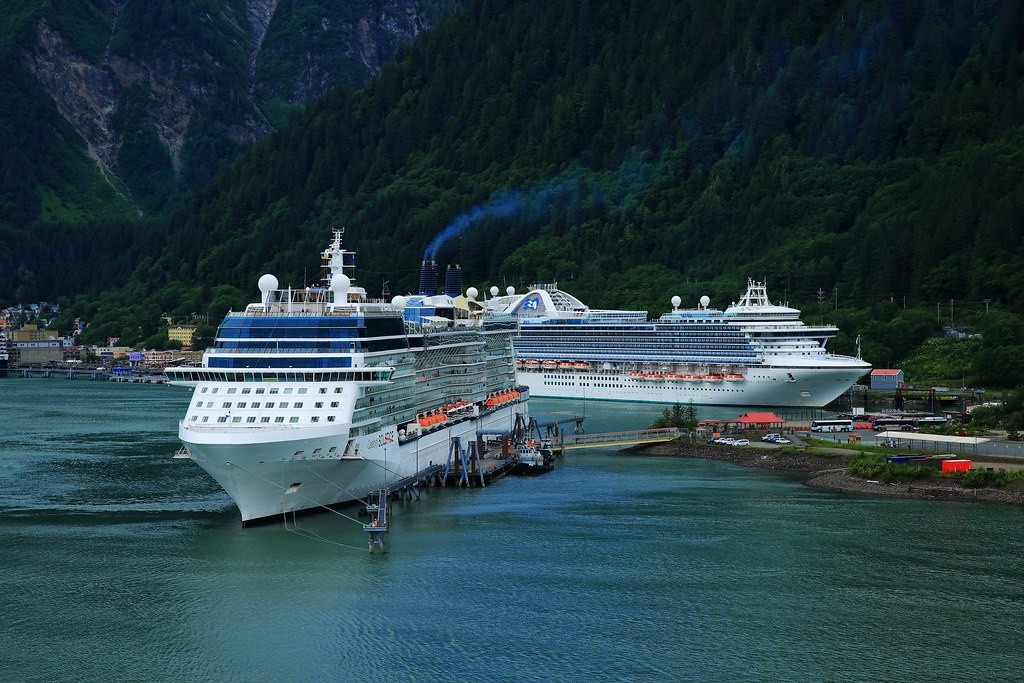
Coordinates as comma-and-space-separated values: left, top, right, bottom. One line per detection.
760, 433, 791, 445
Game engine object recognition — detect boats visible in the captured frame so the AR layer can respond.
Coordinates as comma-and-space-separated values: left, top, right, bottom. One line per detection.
512, 437, 560, 474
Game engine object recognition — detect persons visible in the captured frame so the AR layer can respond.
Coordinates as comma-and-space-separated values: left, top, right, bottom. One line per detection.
374, 518, 378, 526
848, 438, 850, 443
881, 439, 896, 448
834, 435, 835, 441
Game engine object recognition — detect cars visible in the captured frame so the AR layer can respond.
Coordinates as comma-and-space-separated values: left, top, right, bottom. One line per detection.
707, 432, 749, 447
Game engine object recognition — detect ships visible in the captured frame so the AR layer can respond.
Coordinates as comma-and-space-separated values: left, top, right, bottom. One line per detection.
457, 275, 875, 408
163, 229, 529, 528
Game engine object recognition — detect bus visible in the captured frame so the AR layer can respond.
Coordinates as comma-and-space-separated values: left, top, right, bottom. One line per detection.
811, 420, 853, 432
872, 418, 919, 431
